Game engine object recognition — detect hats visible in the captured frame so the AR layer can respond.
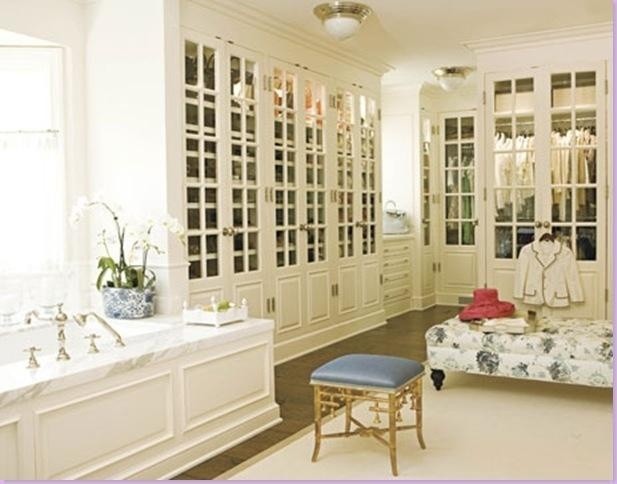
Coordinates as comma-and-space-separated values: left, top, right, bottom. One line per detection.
461, 289, 514, 320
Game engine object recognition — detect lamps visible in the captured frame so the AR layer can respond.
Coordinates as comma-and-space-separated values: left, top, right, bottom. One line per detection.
433, 66, 470, 92
312, 2, 373, 42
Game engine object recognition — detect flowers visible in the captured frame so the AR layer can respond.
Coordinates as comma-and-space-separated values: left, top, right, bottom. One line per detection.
88, 200, 185, 291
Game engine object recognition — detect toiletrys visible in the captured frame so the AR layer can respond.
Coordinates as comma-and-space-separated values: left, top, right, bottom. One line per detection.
53, 302, 68, 322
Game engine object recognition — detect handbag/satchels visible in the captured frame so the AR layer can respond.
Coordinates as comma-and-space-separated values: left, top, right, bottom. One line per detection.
383, 200, 410, 234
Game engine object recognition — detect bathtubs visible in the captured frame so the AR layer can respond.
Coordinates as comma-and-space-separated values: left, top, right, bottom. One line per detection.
0, 313, 123, 368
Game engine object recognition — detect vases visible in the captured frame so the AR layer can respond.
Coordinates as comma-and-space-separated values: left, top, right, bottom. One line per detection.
102, 287, 157, 319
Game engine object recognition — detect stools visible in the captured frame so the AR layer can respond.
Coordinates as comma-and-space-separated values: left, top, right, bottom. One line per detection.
310, 354, 426, 477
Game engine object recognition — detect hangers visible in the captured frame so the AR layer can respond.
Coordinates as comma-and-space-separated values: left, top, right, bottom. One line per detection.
538, 227, 555, 244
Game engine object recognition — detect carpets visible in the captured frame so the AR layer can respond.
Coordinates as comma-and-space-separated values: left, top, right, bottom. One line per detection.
212, 360, 613, 479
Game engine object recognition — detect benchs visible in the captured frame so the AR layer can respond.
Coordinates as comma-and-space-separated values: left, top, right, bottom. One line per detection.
425, 316, 612, 392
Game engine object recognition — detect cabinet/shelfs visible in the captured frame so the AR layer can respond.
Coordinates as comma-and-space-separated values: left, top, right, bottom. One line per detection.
482, 57, 608, 321
383, 233, 415, 318
416, 109, 479, 310
87, 1, 384, 365
